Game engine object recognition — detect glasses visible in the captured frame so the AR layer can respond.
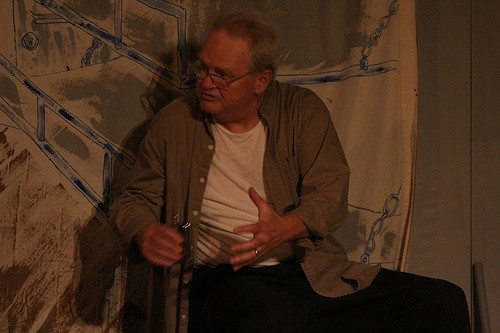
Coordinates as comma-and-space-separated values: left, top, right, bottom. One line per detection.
192, 60, 256, 85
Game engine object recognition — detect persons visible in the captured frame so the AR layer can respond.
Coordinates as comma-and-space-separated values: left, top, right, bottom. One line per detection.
108, 11, 472, 333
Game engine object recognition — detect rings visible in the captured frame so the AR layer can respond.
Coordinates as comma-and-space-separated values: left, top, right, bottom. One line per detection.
255, 249, 259, 256
